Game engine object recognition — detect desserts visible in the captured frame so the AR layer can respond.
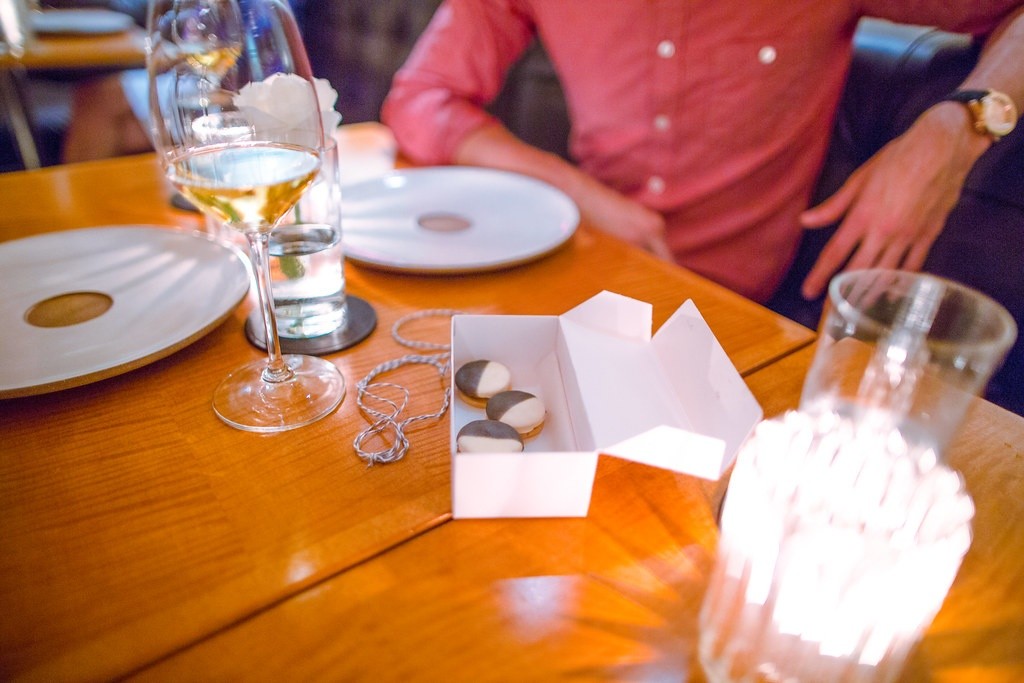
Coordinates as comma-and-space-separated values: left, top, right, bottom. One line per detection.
455, 358, 547, 454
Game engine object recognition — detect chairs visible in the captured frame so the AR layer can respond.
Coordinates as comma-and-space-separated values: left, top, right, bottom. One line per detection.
765, 17, 1024, 418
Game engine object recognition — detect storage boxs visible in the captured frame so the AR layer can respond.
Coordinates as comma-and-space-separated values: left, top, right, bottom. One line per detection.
450, 289, 763, 518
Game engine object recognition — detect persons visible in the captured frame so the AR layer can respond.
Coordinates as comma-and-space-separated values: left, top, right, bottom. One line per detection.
64, 0, 312, 166
380, 0, 1023, 302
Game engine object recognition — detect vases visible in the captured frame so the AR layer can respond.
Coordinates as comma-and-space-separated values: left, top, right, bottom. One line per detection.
234, 131, 350, 339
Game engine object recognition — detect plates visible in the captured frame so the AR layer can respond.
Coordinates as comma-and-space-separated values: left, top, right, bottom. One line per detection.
0, 221, 252, 400
336, 164, 580, 276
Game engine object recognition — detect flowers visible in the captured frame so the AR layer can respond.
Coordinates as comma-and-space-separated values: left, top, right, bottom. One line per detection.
233, 73, 342, 227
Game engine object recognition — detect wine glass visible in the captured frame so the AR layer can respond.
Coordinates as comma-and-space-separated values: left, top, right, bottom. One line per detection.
145, 0, 347, 433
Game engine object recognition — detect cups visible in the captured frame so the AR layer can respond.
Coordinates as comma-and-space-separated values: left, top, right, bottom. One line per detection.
798, 268, 1020, 458
232, 130, 348, 341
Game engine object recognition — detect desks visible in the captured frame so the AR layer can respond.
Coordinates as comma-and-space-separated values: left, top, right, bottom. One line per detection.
0, 121, 1024, 683
0, 0, 179, 171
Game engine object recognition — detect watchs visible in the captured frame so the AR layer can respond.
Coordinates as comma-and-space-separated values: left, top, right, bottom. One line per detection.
942, 88, 1017, 140
